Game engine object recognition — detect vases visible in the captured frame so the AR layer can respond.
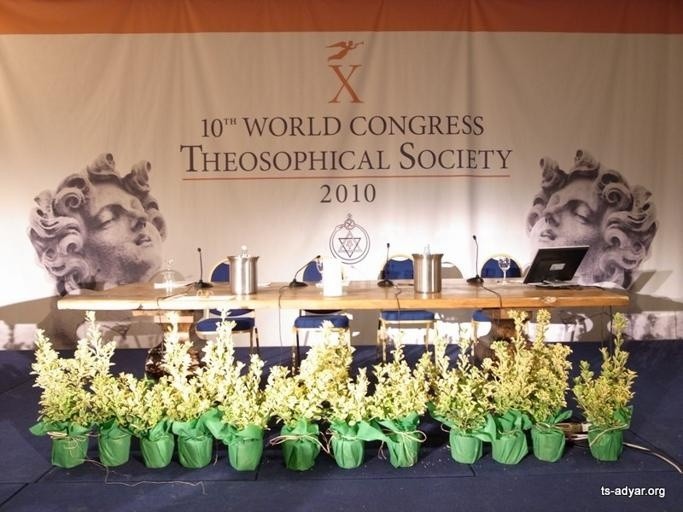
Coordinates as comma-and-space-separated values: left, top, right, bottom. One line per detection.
47, 434, 634, 471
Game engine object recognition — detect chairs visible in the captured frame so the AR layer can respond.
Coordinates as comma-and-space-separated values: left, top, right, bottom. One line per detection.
198, 255, 534, 366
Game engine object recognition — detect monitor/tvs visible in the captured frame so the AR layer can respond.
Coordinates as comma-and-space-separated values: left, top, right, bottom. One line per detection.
523, 246, 590, 284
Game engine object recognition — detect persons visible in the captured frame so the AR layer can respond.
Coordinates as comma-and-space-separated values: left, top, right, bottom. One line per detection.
26, 151, 177, 353
519, 142, 680, 340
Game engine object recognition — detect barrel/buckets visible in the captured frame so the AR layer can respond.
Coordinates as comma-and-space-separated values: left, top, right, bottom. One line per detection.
227, 255, 259, 295
413, 253, 443, 294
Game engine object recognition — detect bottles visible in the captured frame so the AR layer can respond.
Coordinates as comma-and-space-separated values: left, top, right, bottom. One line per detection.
238, 245, 249, 257
422, 244, 431, 256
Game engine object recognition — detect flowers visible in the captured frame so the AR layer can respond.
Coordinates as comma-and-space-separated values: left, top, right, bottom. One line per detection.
27, 320, 639, 432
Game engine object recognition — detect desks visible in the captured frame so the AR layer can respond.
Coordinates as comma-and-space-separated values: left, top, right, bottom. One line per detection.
57, 284, 633, 367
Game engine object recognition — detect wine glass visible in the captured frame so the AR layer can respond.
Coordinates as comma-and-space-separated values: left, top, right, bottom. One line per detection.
162, 256, 177, 296
498, 255, 511, 283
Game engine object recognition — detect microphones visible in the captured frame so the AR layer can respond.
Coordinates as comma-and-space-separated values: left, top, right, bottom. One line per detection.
289, 255, 320, 288
466, 234, 484, 283
378, 243, 393, 287
194, 248, 214, 289
